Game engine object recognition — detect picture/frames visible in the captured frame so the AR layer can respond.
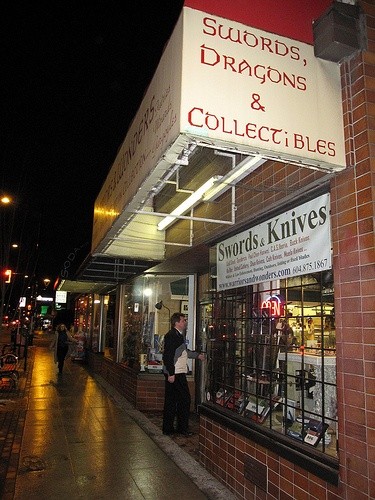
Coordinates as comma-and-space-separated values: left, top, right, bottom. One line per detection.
179, 300, 188, 320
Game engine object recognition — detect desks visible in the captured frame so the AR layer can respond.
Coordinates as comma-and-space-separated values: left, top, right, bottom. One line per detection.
278, 353, 338, 429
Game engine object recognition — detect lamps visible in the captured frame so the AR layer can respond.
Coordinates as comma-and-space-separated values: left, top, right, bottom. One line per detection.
202, 156, 268, 204
157, 175, 225, 233
154, 300, 171, 332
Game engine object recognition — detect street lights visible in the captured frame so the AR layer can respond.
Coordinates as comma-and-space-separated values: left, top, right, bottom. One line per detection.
5, 269, 37, 346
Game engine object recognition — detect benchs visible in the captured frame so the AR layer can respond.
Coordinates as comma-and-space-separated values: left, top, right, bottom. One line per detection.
0, 352, 23, 392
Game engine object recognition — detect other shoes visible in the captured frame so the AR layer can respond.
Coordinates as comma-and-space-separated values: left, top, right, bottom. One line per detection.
58, 368, 63, 374
164, 429, 193, 437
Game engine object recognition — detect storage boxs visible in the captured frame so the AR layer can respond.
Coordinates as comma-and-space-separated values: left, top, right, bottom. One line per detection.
148, 361, 164, 375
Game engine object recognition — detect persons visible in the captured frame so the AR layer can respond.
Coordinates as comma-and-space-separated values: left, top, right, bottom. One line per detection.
161, 312, 206, 437
48, 323, 79, 374
